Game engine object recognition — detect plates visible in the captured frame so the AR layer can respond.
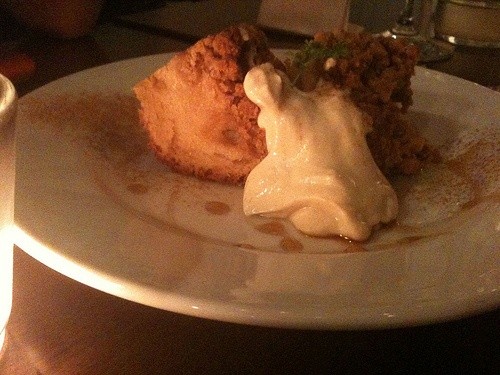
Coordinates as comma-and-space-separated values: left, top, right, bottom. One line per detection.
15, 49, 500, 329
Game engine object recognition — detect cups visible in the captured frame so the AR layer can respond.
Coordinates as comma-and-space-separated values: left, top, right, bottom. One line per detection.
0, 72, 18, 359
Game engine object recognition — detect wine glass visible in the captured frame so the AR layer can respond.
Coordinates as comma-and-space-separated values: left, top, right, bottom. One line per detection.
366, 0, 455, 62
